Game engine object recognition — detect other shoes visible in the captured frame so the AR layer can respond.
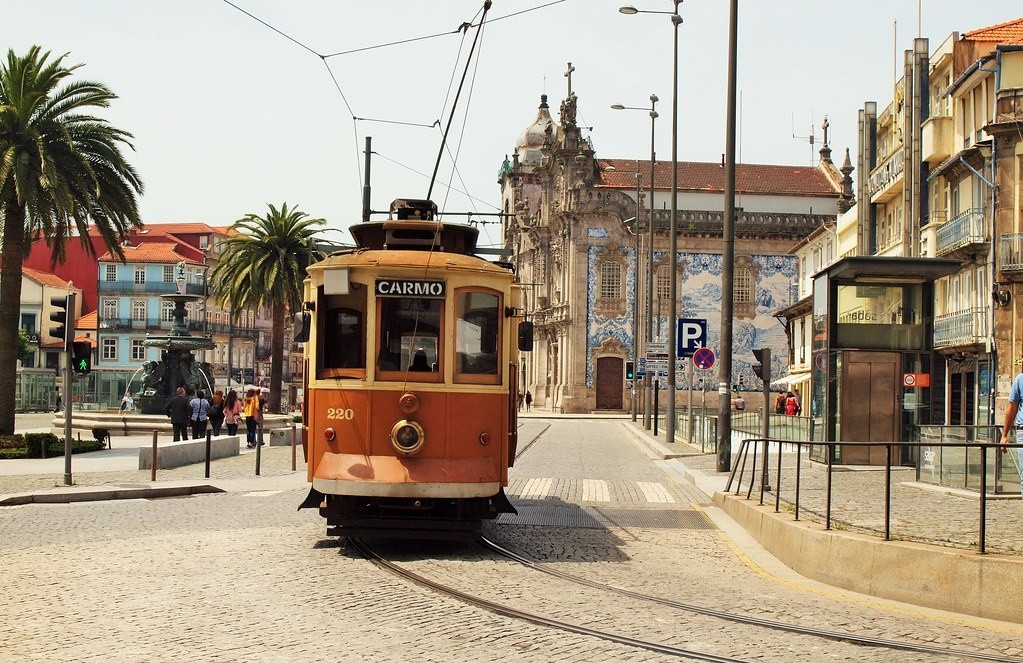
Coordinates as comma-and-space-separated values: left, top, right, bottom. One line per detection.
247, 441, 253, 448
261, 442, 265, 445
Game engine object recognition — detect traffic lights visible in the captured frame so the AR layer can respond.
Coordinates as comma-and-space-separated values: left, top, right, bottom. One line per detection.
752, 347, 771, 383
625, 361, 643, 381
49, 292, 70, 352
72, 340, 91, 374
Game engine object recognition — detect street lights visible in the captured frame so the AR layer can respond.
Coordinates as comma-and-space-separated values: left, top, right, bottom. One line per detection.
617, 0, 685, 443
611, 93, 659, 432
604, 160, 639, 423
621, 216, 645, 357
196, 256, 211, 365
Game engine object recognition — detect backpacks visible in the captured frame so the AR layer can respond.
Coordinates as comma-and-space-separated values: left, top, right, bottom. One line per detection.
206, 400, 222, 418
778, 395, 786, 407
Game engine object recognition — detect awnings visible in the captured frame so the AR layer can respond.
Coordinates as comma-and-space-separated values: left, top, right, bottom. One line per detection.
772, 372, 811, 386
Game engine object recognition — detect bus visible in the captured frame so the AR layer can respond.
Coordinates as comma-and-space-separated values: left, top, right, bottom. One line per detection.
287, 0, 533, 542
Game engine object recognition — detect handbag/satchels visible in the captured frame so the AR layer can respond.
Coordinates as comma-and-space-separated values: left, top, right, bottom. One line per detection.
121, 401, 127, 411
254, 407, 262, 423
794, 406, 798, 414
233, 413, 243, 425
190, 417, 200, 428
221, 417, 227, 429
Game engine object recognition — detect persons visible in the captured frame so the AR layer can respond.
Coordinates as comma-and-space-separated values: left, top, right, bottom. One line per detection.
776, 389, 802, 416
243, 387, 265, 449
165, 387, 193, 443
999, 372, 1023, 500
208, 387, 225, 436
736, 396, 745, 411
117, 392, 133, 415
525, 391, 533, 412
222, 390, 242, 436
517, 390, 523, 412
408, 351, 433, 372
731, 397, 737, 412
190, 391, 210, 439
